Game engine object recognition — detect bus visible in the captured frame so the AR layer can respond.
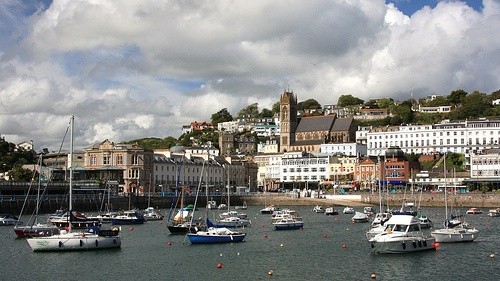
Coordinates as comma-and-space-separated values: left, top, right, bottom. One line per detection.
438, 186, 469, 193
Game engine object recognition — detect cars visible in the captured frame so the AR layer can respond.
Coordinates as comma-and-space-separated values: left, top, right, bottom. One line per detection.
487, 208, 500, 217
466, 208, 483, 214
369, 222, 435, 254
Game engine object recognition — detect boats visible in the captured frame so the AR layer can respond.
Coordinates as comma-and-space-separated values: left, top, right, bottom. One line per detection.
316, 207, 325, 213
343, 206, 355, 214
314, 204, 320, 211
325, 207, 338, 215
364, 207, 376, 216
260, 205, 281, 214
271, 208, 304, 230
352, 213, 369, 223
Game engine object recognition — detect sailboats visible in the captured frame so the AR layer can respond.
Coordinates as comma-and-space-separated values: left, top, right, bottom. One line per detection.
365, 168, 433, 242
0, 114, 252, 252
430, 150, 480, 243
